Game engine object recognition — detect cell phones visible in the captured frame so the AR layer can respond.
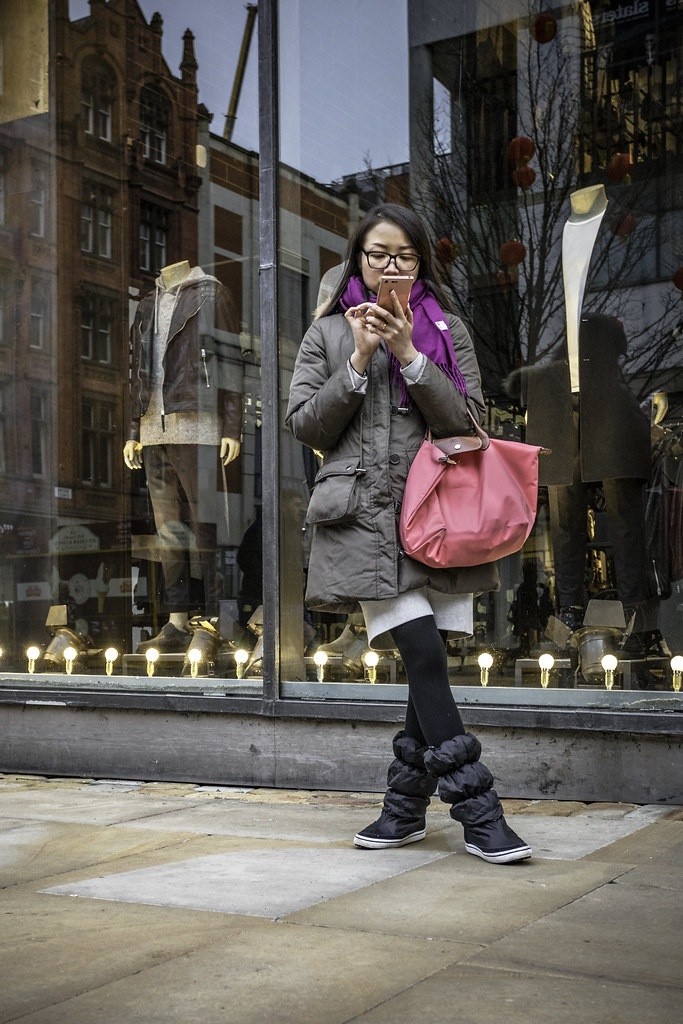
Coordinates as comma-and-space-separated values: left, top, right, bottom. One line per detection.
371, 276, 415, 331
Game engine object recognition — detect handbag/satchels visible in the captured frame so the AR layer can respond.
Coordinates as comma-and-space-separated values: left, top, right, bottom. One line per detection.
398, 407, 552, 569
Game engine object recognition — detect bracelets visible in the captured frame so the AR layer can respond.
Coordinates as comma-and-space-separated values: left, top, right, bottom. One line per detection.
349, 358, 368, 376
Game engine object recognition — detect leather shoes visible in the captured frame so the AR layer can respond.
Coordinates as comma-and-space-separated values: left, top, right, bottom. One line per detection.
136, 621, 194, 655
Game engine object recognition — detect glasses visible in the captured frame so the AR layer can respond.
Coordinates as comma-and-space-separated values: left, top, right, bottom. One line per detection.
360, 246, 422, 272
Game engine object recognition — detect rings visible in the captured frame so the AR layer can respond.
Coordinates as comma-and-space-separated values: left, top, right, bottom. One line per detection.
382, 324, 386, 330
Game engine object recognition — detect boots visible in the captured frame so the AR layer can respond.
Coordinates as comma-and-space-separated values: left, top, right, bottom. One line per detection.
423, 731, 532, 865
353, 730, 439, 850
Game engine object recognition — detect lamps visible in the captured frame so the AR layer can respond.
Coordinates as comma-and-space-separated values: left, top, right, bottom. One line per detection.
314, 651, 328, 682
364, 651, 378, 684
539, 654, 555, 687
478, 652, 493, 687
188, 649, 202, 678
41, 625, 84, 674
600, 654, 618, 689
105, 647, 118, 675
569, 626, 625, 688
145, 648, 159, 677
64, 646, 77, 674
183, 627, 220, 679
26, 647, 40, 674
234, 649, 249, 679
669, 655, 683, 693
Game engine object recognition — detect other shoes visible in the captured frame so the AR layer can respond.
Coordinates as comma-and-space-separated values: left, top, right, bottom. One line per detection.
316, 614, 367, 669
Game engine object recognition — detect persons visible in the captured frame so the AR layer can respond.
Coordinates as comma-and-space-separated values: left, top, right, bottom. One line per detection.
122, 260, 243, 662
522, 184, 670, 687
291, 203, 532, 863
